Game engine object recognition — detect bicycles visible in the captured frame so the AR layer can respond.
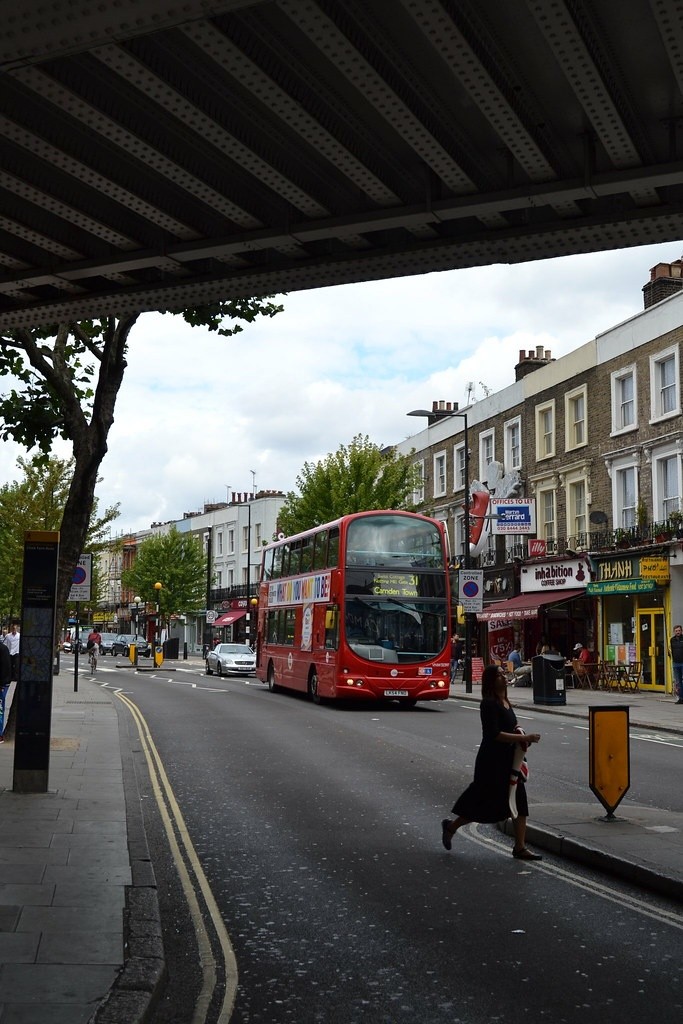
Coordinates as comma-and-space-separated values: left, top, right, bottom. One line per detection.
91, 648, 96, 675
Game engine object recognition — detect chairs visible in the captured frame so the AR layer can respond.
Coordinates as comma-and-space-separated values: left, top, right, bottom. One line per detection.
494, 658, 512, 685
571, 658, 643, 695
506, 661, 529, 687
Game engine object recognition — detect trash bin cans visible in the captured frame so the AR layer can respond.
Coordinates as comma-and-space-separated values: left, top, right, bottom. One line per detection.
162, 637, 179, 659
531, 654, 568, 706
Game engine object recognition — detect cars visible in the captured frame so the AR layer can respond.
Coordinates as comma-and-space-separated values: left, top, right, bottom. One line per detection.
99, 633, 119, 656
205, 643, 257, 677
111, 633, 151, 658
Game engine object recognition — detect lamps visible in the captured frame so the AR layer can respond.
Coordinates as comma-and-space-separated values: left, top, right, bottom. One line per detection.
566, 548, 578, 557
513, 555, 525, 564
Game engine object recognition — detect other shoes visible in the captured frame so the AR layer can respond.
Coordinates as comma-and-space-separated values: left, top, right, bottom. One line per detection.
513, 845, 543, 860
442, 818, 453, 851
675, 699, 683, 704
0, 736, 4, 744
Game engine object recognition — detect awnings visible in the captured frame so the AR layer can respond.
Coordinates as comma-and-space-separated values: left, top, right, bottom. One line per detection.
212, 611, 246, 625
476, 590, 586, 622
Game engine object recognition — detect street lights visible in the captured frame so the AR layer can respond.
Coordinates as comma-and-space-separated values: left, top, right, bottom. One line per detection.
224, 499, 253, 644
251, 599, 258, 639
404, 409, 503, 693
153, 582, 162, 668
134, 597, 142, 641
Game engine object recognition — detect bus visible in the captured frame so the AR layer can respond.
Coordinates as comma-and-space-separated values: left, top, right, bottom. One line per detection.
257, 510, 466, 708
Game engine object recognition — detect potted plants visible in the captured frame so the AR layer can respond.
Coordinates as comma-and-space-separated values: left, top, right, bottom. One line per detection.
614, 528, 630, 548
653, 519, 674, 543
641, 529, 653, 545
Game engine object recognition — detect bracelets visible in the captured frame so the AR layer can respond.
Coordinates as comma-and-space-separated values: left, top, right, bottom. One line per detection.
514, 726, 520, 732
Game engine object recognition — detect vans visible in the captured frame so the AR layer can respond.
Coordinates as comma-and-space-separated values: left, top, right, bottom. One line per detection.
70, 631, 92, 654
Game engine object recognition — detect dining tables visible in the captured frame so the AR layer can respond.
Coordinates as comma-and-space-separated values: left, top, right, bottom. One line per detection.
521, 660, 572, 666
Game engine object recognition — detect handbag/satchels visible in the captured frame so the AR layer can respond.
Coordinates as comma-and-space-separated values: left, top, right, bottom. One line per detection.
87, 640, 94, 649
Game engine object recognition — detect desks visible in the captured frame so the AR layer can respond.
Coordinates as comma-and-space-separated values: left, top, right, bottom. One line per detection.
607, 664, 633, 693
579, 663, 602, 690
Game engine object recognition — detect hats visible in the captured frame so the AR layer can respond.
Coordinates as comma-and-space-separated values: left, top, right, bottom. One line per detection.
573, 643, 582, 650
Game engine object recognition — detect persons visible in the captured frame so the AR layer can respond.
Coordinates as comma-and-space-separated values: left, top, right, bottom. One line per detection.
88, 628, 102, 670
0, 625, 20, 744
202, 636, 220, 659
670, 625, 683, 704
508, 643, 533, 684
450, 633, 466, 684
571, 642, 594, 675
441, 664, 543, 861
536, 634, 561, 656
